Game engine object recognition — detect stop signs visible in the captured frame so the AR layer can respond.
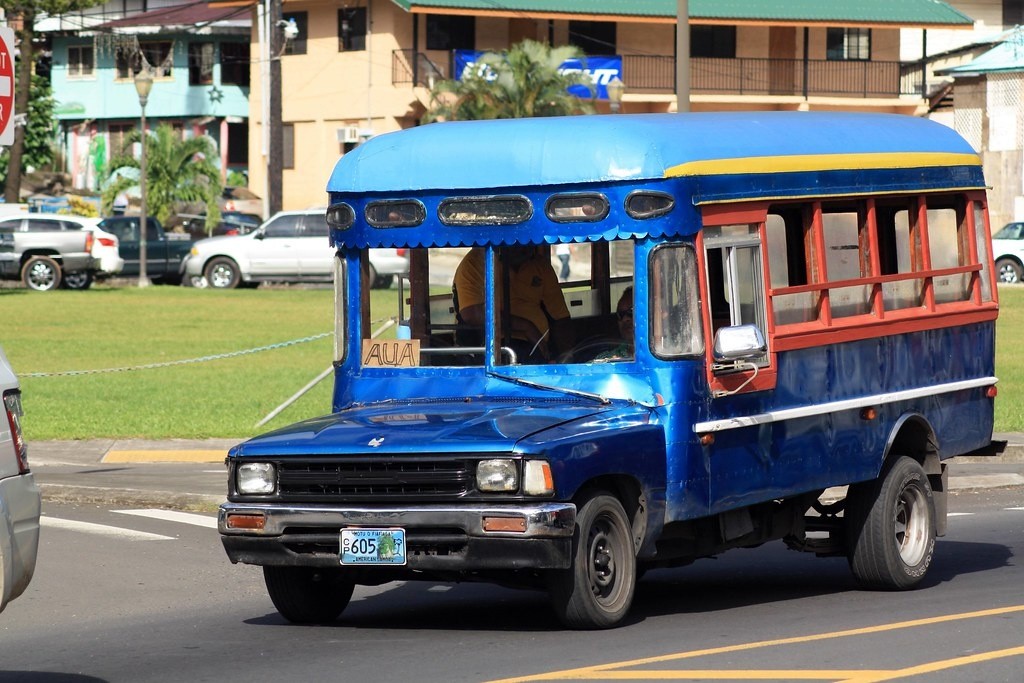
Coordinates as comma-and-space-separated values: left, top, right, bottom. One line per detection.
0, 25, 16, 146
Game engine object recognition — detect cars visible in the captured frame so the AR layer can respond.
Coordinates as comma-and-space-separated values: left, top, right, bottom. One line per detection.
990, 221, 1024, 284
0, 356, 43, 614
184, 211, 263, 244
0, 218, 102, 292
0, 210, 125, 292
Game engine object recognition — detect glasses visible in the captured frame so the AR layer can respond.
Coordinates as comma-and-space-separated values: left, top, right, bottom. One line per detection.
615, 307, 633, 320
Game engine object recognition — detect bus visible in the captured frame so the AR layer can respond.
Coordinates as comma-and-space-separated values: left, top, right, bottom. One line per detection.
212, 112, 1007, 633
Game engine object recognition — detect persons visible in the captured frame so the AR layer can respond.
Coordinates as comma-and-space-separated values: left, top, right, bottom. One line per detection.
555, 243, 578, 284
595, 286, 635, 360
450, 245, 574, 364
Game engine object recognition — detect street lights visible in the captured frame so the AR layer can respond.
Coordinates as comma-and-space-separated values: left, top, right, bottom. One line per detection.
132, 70, 157, 288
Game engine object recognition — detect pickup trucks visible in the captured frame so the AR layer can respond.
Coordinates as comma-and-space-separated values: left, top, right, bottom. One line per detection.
62, 215, 201, 291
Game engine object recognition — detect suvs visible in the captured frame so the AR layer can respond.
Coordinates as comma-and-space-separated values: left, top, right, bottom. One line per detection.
179, 207, 411, 291
